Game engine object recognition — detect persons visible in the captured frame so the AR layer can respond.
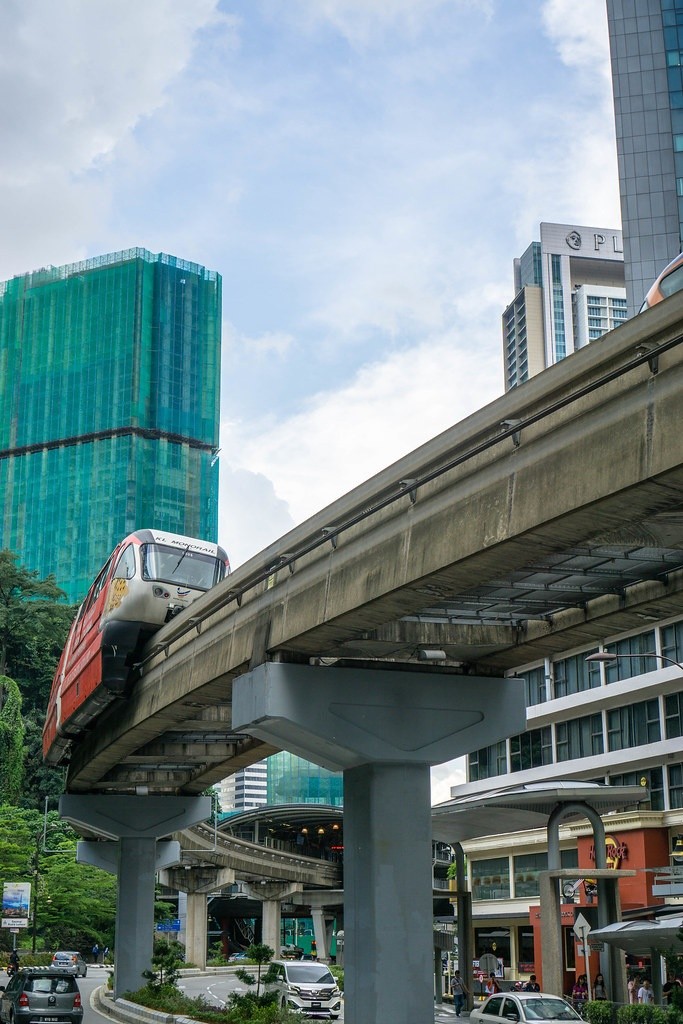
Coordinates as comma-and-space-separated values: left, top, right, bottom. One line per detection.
627, 972, 654, 1004
662, 969, 683, 1005
92, 944, 98, 964
10, 948, 20, 972
104, 948, 109, 959
512, 975, 540, 992
487, 972, 502, 997
451, 970, 470, 1017
572, 973, 608, 1014
488, 942, 504, 958
297, 949, 304, 960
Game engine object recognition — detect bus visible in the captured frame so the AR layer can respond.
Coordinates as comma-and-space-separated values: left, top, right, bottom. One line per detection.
246, 917, 337, 963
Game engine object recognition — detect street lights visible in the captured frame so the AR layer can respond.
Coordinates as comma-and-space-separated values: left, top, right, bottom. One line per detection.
32, 895, 54, 956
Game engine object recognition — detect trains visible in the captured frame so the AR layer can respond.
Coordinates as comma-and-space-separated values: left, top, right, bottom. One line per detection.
41, 526, 231, 768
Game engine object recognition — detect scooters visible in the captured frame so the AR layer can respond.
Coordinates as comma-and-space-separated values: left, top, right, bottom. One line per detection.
7, 960, 20, 977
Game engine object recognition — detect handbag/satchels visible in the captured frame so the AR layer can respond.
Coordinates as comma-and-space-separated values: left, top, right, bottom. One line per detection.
463, 992, 467, 999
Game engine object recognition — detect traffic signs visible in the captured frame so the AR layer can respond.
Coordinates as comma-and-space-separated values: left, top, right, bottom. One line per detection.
156, 919, 181, 932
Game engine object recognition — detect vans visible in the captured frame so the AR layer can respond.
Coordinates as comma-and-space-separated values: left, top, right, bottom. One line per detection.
264, 958, 342, 1019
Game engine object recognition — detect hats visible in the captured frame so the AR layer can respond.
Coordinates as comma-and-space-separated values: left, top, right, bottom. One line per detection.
13, 949, 17, 952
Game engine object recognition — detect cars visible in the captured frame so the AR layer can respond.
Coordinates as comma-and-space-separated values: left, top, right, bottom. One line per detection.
0, 965, 84, 1024
468, 991, 592, 1024
50, 951, 88, 979
228, 953, 251, 963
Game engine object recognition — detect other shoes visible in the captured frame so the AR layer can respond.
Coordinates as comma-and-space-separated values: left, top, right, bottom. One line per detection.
459, 1009, 461, 1013
456, 1013, 460, 1017
